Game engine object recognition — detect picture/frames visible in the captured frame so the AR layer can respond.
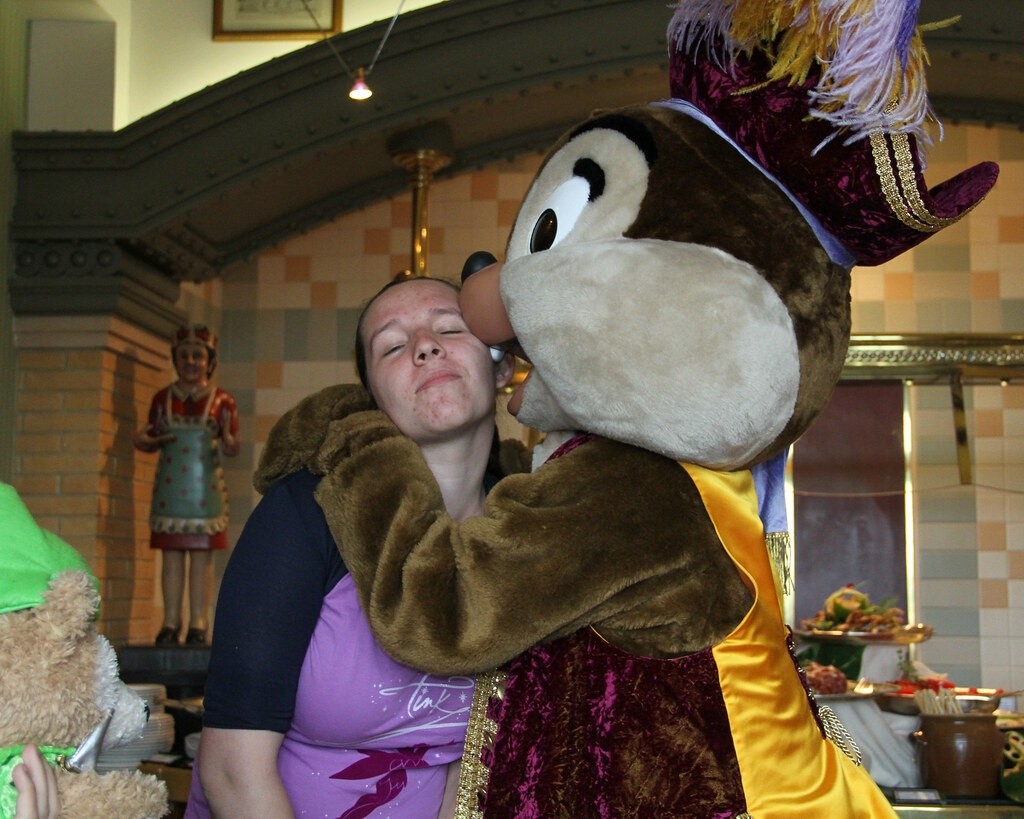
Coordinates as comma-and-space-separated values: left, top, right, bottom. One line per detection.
211, 0, 345, 44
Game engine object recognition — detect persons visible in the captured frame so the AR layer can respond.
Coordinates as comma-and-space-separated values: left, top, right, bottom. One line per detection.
132, 322, 243, 649
185, 272, 514, 819
12, 743, 62, 819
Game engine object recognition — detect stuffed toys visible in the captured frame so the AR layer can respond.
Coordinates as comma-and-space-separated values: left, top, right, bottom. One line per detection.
0, 482, 170, 819
254, 0, 999, 819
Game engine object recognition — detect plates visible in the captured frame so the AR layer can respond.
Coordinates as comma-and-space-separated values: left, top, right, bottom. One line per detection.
813, 681, 902, 700
876, 691, 1000, 715
792, 630, 937, 646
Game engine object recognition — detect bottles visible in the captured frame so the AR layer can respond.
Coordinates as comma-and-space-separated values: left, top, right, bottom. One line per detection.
909, 715, 1005, 798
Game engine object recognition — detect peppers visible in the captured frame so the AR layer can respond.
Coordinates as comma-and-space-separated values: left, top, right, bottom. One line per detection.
891, 677, 979, 694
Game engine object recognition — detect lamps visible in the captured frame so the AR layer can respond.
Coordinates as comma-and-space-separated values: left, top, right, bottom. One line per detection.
300, 0, 407, 101
